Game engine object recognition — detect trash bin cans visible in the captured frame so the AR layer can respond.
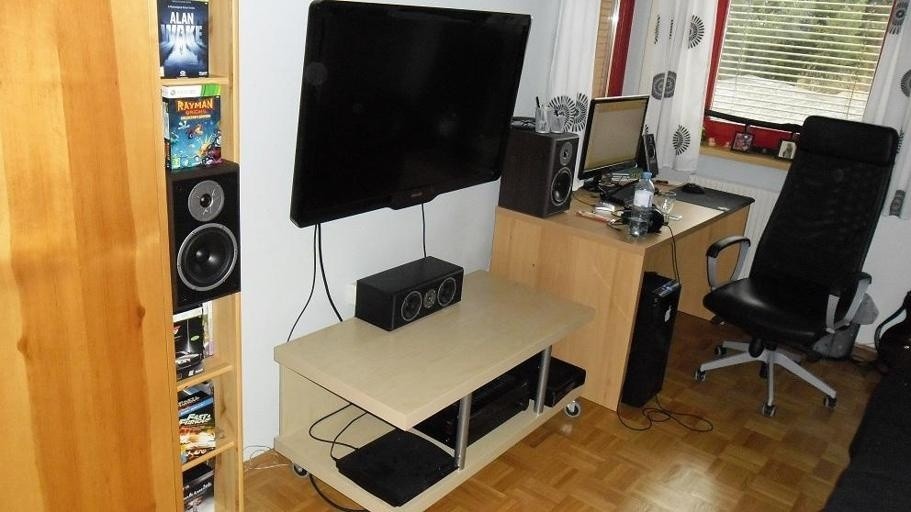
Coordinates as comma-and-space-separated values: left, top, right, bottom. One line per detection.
814, 296, 880, 358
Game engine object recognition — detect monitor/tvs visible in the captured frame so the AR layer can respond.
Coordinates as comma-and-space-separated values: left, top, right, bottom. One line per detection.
578, 94, 649, 197
290, 0, 531, 229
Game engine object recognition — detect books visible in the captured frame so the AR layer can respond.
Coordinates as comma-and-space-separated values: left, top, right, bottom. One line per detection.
172, 300, 221, 512
160, 84, 224, 171
156, 0, 212, 79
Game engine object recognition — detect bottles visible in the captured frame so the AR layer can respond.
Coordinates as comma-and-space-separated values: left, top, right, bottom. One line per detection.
627, 172, 655, 238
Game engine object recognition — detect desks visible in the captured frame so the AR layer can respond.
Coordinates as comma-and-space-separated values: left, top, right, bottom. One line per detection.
489, 178, 756, 412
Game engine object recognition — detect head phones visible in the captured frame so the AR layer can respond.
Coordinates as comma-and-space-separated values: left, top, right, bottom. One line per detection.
607, 208, 664, 234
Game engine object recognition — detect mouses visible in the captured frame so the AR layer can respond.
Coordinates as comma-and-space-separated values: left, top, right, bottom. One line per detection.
681, 183, 705, 195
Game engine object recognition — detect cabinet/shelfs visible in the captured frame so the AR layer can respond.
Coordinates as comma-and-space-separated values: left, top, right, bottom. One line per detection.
151, 0, 244, 512
274, 269, 595, 512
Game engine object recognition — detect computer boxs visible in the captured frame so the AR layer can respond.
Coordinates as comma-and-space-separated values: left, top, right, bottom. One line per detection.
621, 271, 681, 408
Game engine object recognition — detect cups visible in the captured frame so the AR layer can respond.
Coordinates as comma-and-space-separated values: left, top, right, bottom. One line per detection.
535, 106, 554, 134
551, 113, 566, 134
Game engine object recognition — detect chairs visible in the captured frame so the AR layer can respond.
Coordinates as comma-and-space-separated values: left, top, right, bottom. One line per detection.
693, 116, 900, 418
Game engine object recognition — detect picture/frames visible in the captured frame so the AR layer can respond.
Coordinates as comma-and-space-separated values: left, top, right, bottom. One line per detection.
775, 138, 798, 161
731, 131, 755, 153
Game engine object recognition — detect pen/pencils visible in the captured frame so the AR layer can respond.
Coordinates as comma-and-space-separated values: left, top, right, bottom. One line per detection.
554, 111, 560, 118
534, 97, 542, 108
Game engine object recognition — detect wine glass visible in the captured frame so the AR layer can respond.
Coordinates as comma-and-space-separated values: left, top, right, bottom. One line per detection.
657, 189, 676, 228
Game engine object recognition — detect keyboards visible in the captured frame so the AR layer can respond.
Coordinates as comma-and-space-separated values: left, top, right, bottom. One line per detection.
600, 181, 659, 206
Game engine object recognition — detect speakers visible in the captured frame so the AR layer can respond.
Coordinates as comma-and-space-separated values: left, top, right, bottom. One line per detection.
636, 134, 658, 178
166, 158, 240, 313
498, 125, 579, 218
355, 256, 464, 331
